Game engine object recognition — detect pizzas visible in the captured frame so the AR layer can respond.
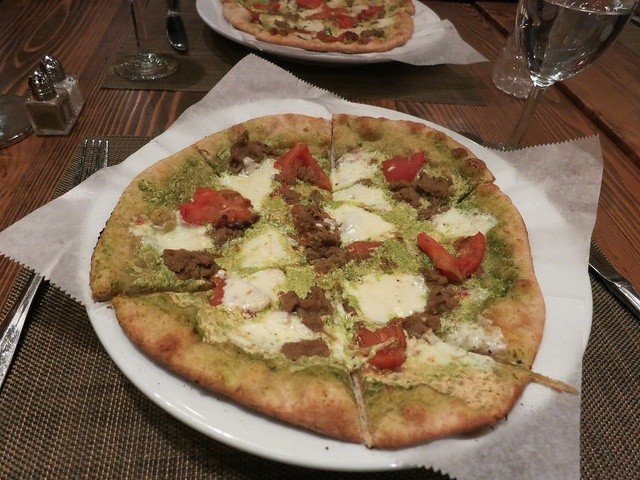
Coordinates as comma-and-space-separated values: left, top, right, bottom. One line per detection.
93, 113, 546, 449
221, 2, 417, 52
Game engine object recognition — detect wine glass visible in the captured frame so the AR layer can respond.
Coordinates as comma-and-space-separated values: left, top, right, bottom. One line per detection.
457, 1, 616, 147
114, 1, 179, 82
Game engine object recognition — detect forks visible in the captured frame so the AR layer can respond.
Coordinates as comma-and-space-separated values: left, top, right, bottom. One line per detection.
0, 139, 110, 384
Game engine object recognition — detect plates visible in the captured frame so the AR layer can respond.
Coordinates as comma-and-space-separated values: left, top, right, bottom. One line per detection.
195, 2, 445, 64
83, 99, 576, 473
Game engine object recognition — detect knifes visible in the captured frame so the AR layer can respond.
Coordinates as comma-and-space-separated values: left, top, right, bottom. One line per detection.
160, 0, 189, 53
588, 244, 640, 320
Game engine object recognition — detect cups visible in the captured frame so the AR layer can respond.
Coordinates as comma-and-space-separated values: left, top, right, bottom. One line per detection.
492, 30, 534, 100
